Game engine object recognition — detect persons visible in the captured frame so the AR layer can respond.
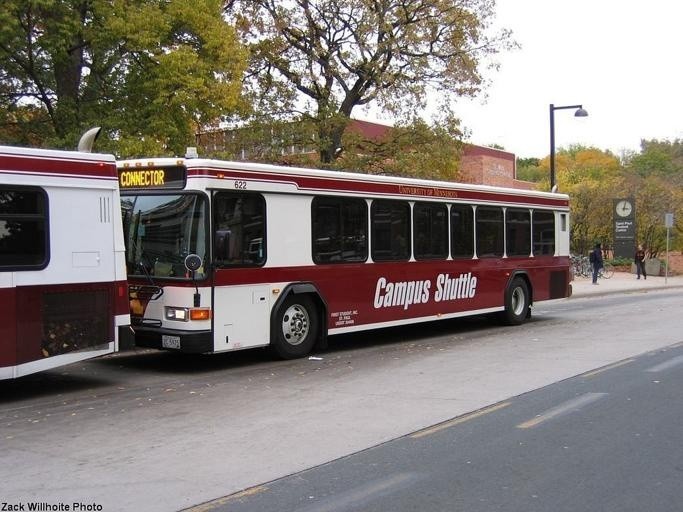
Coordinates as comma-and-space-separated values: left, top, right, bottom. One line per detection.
592, 243, 603, 284
635, 244, 646, 279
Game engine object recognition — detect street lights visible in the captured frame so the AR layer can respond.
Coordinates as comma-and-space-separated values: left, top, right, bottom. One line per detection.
549, 100, 588, 193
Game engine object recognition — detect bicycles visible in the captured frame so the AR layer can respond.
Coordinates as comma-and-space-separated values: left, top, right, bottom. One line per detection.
569, 253, 614, 281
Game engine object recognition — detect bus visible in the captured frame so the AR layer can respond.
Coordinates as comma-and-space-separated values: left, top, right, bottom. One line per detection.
0, 145, 135, 384
117, 158, 578, 361
